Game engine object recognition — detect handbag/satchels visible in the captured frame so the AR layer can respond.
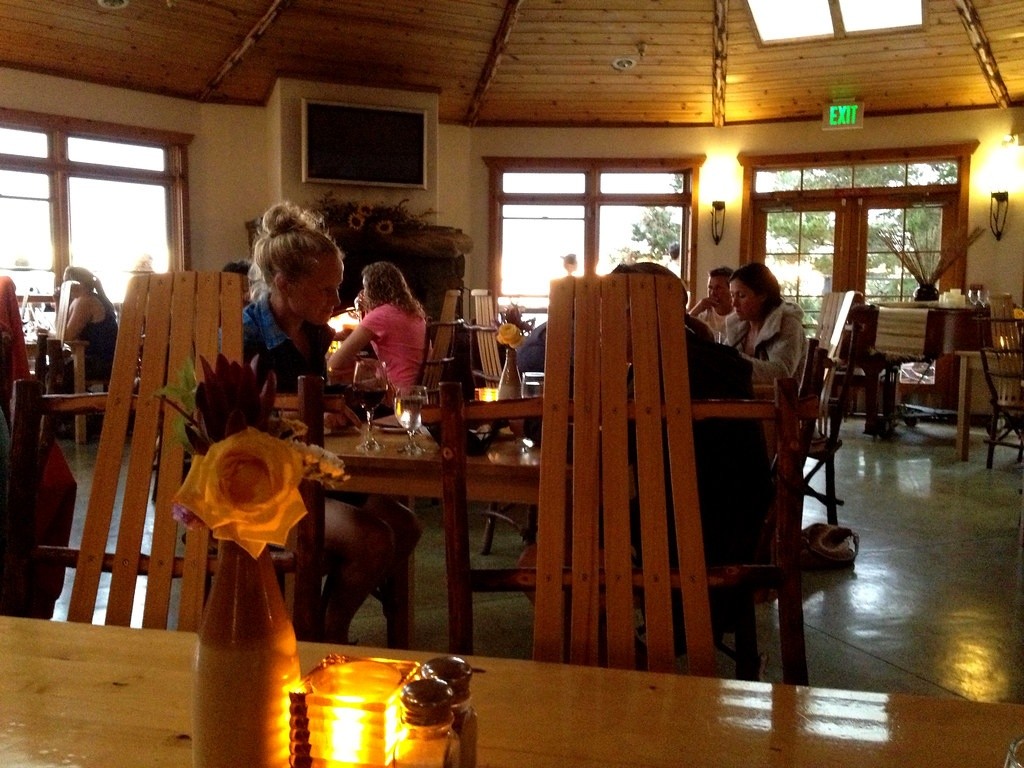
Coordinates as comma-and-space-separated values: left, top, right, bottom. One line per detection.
800, 522, 860, 572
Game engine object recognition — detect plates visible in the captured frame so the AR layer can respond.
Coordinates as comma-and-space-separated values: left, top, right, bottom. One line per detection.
371, 420, 408, 432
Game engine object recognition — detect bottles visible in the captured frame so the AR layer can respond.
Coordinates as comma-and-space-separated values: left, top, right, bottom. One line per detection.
394, 657, 477, 768
394, 385, 429, 456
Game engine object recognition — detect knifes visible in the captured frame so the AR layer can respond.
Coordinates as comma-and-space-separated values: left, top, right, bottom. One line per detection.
362, 421, 399, 427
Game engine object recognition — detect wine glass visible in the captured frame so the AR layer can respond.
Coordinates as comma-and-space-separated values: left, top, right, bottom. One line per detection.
353, 358, 388, 452
977, 290, 989, 307
346, 290, 370, 320
19, 307, 30, 336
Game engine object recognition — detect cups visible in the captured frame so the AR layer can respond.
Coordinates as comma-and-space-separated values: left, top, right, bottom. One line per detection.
36, 326, 50, 338
521, 371, 544, 398
968, 289, 978, 305
712, 331, 721, 343
939, 289, 965, 307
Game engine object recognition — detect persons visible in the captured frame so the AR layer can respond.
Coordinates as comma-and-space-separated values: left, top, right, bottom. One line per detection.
328, 261, 427, 418
719, 260, 809, 399
513, 263, 781, 651
241, 202, 423, 649
216, 262, 254, 331
51, 264, 117, 438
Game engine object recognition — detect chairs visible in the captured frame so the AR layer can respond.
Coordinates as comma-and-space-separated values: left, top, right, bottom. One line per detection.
0, 270, 1024, 679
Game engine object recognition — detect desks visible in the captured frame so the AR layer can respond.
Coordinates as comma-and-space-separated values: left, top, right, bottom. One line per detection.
1, 612, 1024, 768
953, 347, 1024, 462
297, 383, 637, 520
17, 322, 89, 447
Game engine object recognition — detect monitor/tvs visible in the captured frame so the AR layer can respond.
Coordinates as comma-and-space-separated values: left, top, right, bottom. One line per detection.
300, 98, 428, 190
